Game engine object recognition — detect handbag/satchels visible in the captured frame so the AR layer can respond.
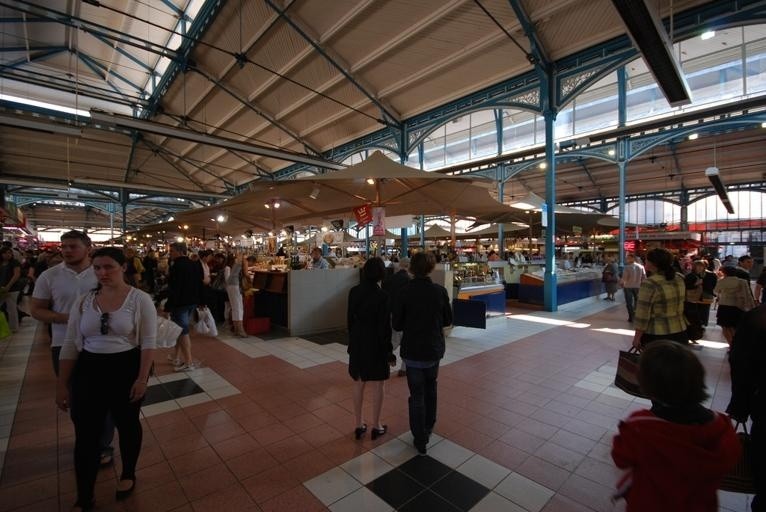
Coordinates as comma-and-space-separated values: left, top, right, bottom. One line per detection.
602, 271, 618, 283
717, 432, 759, 494
686, 285, 703, 302
240, 275, 251, 290
615, 351, 651, 399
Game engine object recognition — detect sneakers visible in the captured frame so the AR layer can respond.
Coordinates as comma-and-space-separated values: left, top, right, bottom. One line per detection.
115, 474, 136, 500
604, 298, 615, 301
70, 498, 96, 512
100, 453, 111, 465
168, 358, 193, 371
413, 435, 429, 454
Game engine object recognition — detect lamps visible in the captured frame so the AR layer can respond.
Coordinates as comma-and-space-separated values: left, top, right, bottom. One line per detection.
309, 180, 321, 200
609, 0, 692, 108
704, 135, 735, 214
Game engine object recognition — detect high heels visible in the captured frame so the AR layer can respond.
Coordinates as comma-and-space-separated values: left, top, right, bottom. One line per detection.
355, 424, 367, 439
371, 425, 387, 440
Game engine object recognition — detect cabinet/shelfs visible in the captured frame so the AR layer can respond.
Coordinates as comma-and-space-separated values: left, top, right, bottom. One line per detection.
251, 271, 288, 328
453, 261, 605, 317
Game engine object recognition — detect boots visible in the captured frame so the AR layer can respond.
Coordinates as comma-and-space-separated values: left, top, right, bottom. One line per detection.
233, 321, 248, 338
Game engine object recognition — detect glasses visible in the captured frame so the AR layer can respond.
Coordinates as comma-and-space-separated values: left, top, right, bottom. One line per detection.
100, 313, 109, 335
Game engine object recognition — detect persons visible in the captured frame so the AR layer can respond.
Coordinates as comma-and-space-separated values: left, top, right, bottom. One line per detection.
381, 255, 413, 377
560, 244, 766, 354
31, 228, 116, 468
724, 305, 765, 511
0, 236, 257, 339
56, 247, 158, 512
481, 248, 531, 263
158, 242, 206, 373
392, 252, 452, 454
610, 338, 742, 511
346, 256, 392, 440
